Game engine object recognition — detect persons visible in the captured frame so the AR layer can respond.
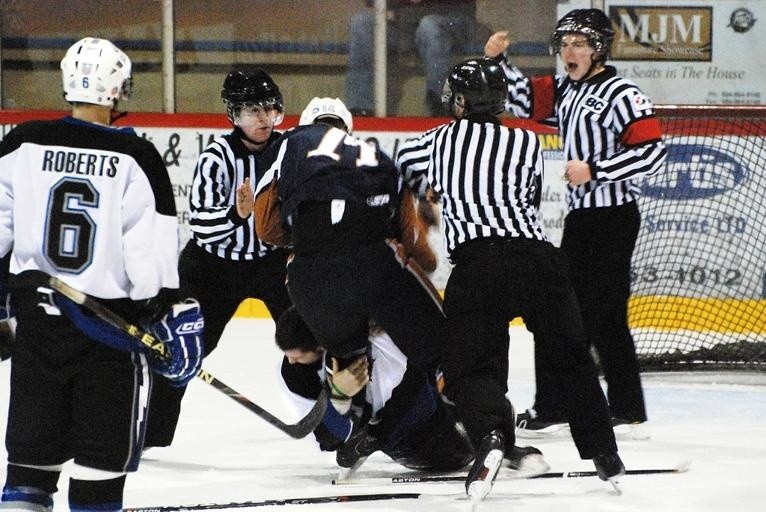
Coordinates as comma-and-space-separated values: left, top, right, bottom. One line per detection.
0, 36, 207, 512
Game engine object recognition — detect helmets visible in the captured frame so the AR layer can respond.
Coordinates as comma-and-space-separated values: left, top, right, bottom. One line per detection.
61, 36, 132, 110
220, 67, 285, 127
298, 96, 354, 134
549, 8, 616, 65
442, 56, 509, 114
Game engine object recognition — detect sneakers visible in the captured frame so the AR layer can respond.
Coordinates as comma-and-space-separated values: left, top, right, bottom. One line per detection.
515, 409, 554, 430
592, 453, 626, 481
466, 431, 504, 498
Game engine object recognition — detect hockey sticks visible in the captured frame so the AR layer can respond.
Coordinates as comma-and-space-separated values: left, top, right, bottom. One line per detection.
332, 432, 706, 487
48, 277, 330, 439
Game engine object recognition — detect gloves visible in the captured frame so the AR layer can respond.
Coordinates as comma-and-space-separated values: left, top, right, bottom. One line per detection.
144, 298, 204, 385
